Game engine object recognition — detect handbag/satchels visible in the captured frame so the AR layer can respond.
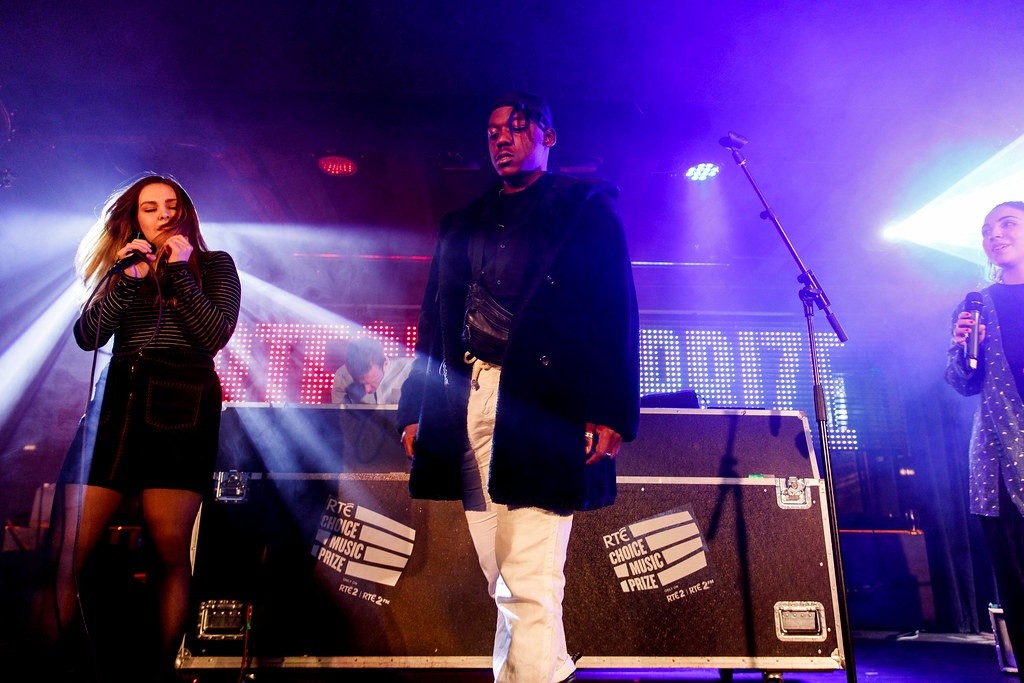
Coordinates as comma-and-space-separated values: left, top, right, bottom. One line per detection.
461, 282, 513, 366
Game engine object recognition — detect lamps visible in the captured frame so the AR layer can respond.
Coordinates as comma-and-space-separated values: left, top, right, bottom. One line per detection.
671, 155, 727, 187
307, 140, 369, 177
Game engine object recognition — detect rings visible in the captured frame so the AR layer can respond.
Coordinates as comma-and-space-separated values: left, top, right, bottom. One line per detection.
398, 430, 405, 442
585, 432, 593, 439
606, 451, 612, 456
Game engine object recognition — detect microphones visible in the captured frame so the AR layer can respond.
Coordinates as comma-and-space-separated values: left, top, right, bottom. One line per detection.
108, 242, 157, 278
965, 292, 984, 371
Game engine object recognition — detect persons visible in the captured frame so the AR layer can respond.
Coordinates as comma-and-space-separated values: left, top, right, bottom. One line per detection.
945, 201, 1024, 683
395, 85, 640, 683
332, 337, 420, 475
29, 174, 242, 683
